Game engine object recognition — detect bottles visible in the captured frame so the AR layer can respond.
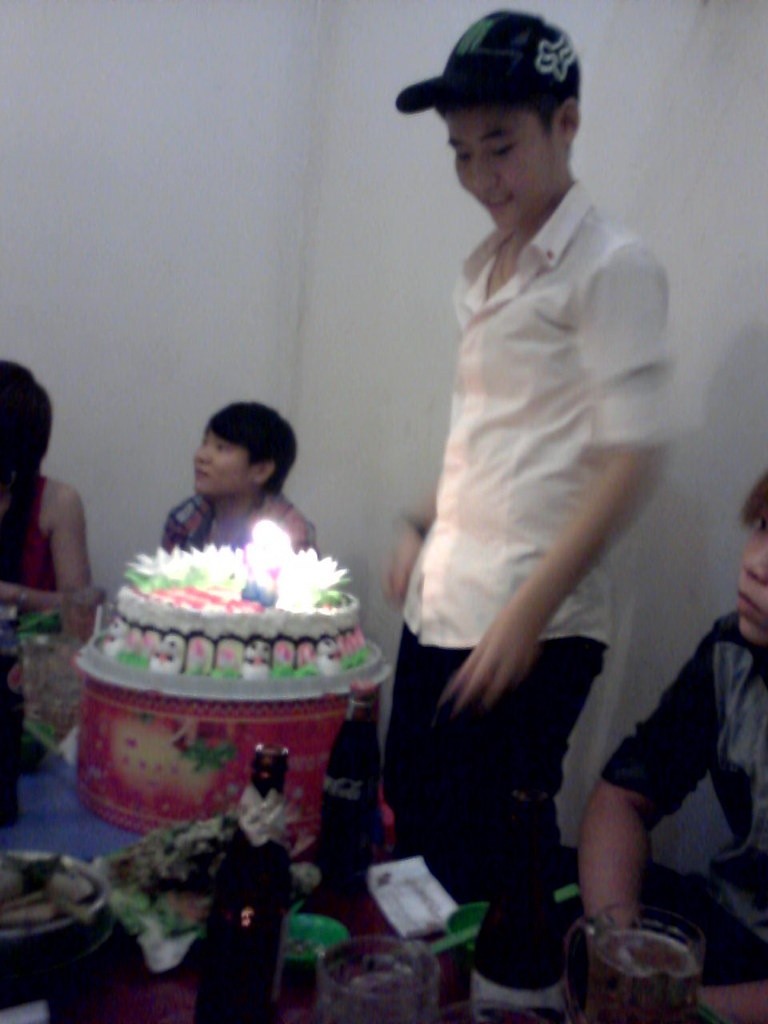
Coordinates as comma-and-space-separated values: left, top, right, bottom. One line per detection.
193, 744, 293, 1024
0, 605, 27, 831
315, 680, 384, 904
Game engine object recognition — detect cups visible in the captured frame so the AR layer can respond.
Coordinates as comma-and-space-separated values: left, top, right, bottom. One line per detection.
318, 937, 444, 1024
562, 903, 705, 1024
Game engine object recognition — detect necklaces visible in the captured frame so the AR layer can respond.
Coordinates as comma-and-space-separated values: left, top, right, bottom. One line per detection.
500, 239, 510, 284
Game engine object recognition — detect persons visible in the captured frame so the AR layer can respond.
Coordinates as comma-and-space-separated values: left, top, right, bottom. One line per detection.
575, 470, 768, 1024
378, 12, 677, 851
0, 359, 101, 616
160, 401, 322, 561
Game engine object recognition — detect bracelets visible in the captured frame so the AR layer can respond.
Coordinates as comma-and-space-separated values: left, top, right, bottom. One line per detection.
17, 586, 29, 609
401, 509, 428, 540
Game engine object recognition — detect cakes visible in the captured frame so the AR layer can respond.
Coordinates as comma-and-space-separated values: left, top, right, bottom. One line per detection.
85, 543, 385, 692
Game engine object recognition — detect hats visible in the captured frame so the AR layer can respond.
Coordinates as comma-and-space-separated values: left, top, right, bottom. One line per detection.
395, 12, 579, 115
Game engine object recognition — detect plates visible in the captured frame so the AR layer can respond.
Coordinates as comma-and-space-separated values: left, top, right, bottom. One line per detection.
285, 910, 347, 960
0, 846, 112, 972
